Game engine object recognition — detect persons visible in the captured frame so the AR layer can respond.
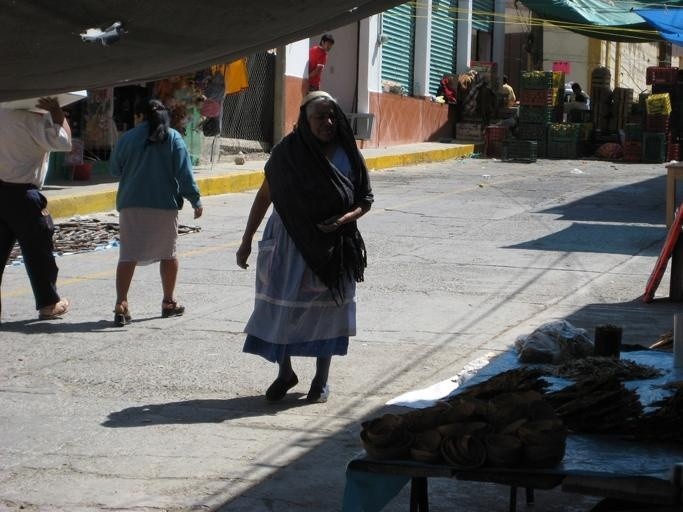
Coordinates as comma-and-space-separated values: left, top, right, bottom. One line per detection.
502, 75, 516, 107
109, 98, 205, 327
235, 90, 375, 404
571, 94, 589, 110
570, 83, 590, 102
0, 96, 73, 319
309, 34, 334, 90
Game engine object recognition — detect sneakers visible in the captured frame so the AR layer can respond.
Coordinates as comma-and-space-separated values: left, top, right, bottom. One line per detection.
307, 378, 329, 403
265, 372, 297, 401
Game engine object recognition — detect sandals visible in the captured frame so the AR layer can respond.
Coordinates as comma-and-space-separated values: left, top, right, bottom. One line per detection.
37, 295, 70, 319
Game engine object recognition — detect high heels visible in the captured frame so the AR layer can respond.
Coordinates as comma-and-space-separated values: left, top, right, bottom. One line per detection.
113, 302, 131, 327
160, 299, 185, 317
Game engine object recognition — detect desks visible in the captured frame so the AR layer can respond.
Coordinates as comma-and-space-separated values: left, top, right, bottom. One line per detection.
348, 302, 681, 512
